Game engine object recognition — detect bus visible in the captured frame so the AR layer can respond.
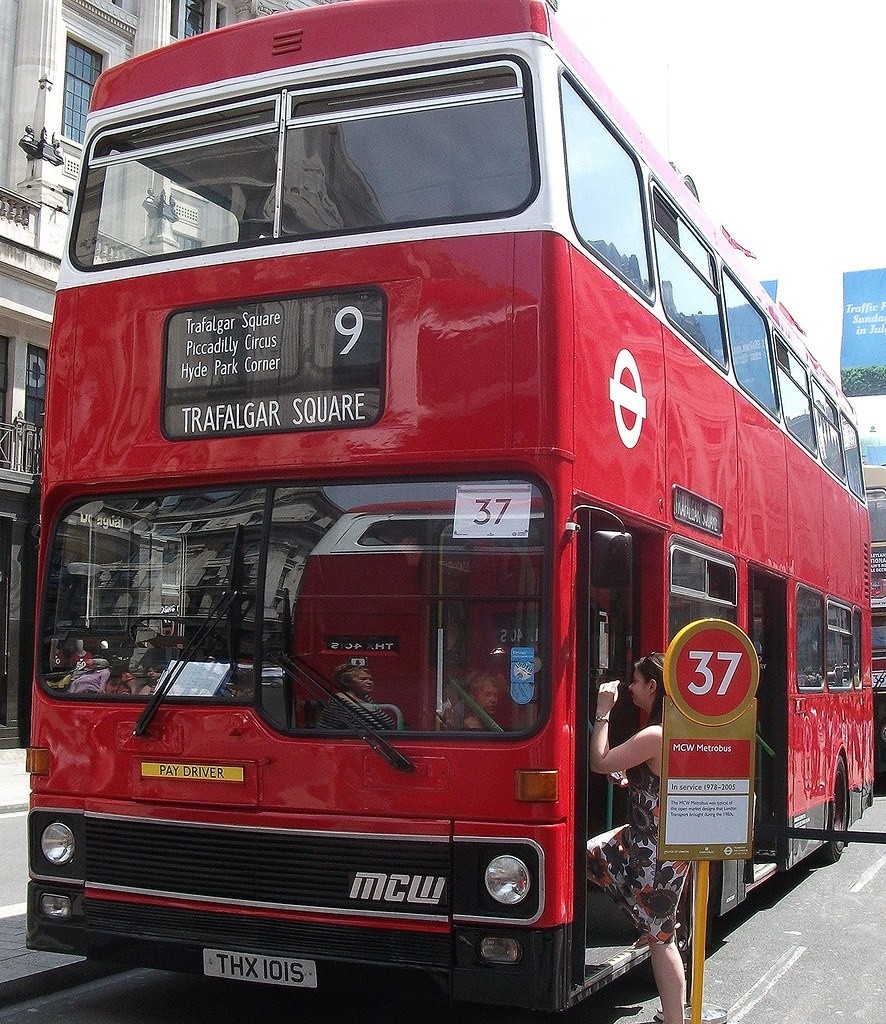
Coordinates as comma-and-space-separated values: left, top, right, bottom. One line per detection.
754, 462, 885, 794
22, 1, 877, 1015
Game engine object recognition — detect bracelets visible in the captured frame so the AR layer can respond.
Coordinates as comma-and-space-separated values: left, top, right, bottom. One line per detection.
595, 716, 609, 723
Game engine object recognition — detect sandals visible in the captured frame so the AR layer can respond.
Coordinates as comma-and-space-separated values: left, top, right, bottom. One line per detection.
633, 923, 681, 949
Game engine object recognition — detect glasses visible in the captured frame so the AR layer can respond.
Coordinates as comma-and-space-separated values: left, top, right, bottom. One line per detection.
644, 652, 657, 673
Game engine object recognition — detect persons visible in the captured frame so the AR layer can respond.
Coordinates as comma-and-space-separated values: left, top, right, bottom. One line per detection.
138, 665, 165, 695
461, 675, 506, 733
51, 638, 110, 669
585, 653, 692, 1024
318, 665, 397, 733
